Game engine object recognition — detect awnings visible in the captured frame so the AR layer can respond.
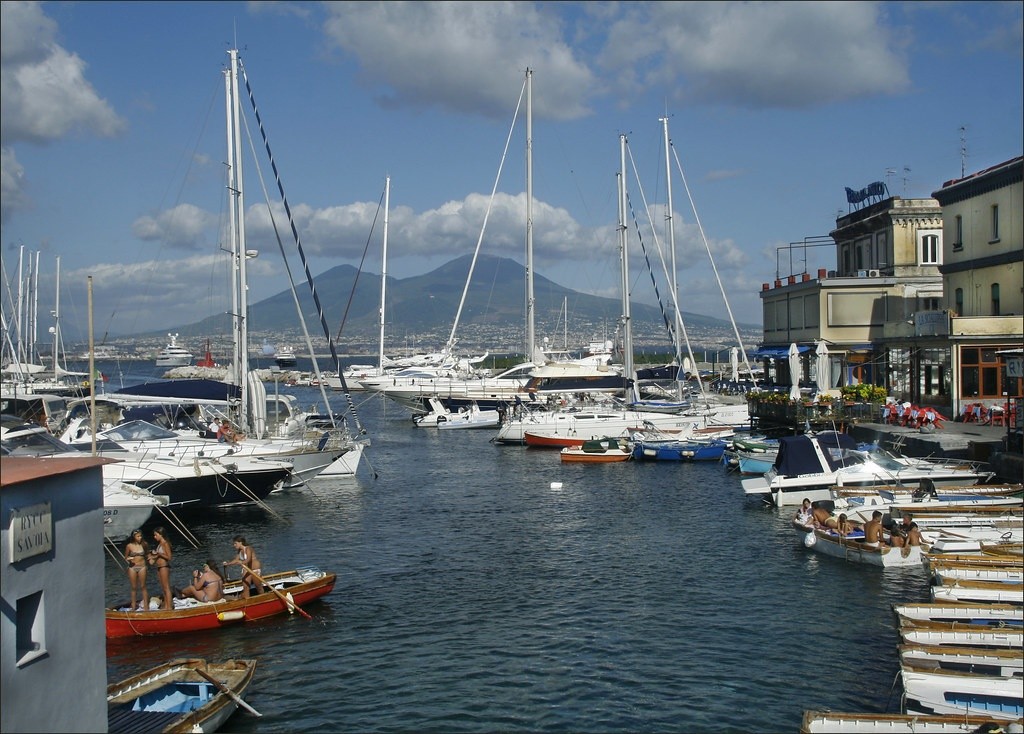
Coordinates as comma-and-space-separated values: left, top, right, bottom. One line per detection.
746, 346, 812, 359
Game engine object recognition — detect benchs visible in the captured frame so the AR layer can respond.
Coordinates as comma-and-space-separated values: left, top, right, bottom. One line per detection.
108, 669, 246, 704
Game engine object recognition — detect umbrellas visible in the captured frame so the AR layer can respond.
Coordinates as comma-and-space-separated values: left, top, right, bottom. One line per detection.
788, 343, 801, 400
813, 342, 829, 404
730, 346, 739, 383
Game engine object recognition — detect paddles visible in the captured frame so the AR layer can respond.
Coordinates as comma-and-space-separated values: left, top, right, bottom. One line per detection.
855, 509, 869, 525
194, 666, 262, 718
240, 562, 313, 619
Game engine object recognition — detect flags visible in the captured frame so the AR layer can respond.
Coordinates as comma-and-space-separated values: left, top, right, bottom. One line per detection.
93, 368, 110, 382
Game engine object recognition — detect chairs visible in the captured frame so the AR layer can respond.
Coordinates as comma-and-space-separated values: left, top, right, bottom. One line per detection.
882, 401, 950, 430
962, 403, 1017, 428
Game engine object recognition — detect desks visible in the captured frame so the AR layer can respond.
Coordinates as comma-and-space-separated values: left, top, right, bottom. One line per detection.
833, 527, 866, 538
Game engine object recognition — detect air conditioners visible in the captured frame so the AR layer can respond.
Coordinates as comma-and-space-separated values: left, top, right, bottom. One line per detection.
858, 270, 880, 278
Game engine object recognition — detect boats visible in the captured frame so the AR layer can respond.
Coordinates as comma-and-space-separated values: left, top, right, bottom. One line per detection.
896, 643, 1023, 675
897, 625, 1024, 650
155, 332, 193, 366
106, 566, 337, 646
901, 690, 1024, 720
272, 345, 298, 367
928, 586, 1024, 606
889, 601, 1024, 628
899, 667, 1024, 712
801, 709, 1024, 734
559, 437, 631, 462
107, 656, 259, 734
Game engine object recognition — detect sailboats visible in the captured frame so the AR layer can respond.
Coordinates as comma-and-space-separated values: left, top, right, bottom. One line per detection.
2, 45, 1024, 602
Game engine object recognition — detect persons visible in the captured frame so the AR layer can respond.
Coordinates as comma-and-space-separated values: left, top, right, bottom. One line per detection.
792, 498, 814, 525
124, 529, 150, 611
225, 536, 264, 598
890, 513, 931, 548
209, 417, 220, 432
148, 527, 174, 610
811, 501, 864, 531
864, 510, 885, 547
174, 560, 223, 603
218, 420, 237, 444
832, 514, 853, 537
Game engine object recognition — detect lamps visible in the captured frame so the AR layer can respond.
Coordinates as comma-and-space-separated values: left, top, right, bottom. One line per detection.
906, 320, 914, 325
